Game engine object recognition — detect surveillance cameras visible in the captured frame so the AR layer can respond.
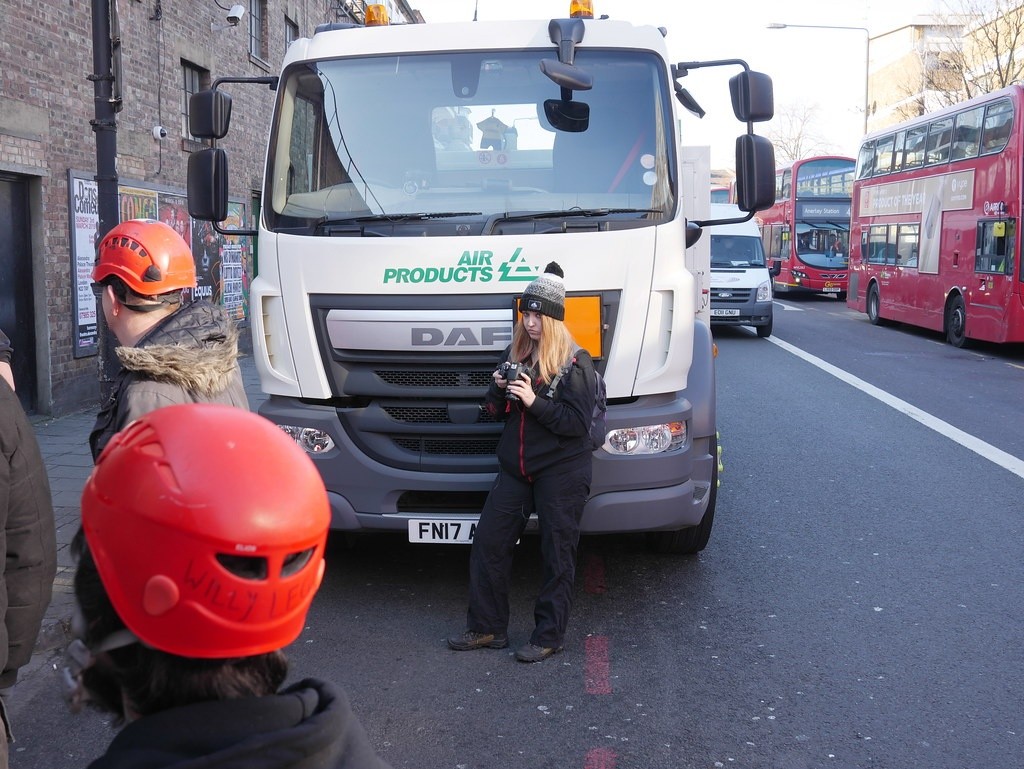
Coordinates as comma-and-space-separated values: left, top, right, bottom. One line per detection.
154, 126, 166, 140
226, 4, 245, 23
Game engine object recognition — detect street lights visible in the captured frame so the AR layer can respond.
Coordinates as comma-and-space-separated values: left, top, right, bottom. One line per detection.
765, 22, 871, 143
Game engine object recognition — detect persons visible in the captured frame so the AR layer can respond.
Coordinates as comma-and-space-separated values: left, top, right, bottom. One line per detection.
74, 217, 251, 464
0, 326, 21, 398
444, 272, 600, 663
198, 244, 211, 274
768, 224, 847, 255
0, 360, 61, 769
60, 403, 396, 769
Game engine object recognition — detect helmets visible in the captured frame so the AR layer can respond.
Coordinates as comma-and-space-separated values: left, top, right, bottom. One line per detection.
80, 403, 331, 662
91, 219, 196, 295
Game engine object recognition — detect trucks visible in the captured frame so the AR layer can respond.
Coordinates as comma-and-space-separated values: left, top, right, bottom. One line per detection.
181, 0, 780, 556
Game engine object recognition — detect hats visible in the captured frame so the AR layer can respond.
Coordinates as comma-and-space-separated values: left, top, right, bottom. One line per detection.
518, 261, 566, 322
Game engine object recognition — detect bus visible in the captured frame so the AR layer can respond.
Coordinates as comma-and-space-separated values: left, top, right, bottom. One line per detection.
707, 156, 857, 301
848, 85, 1024, 350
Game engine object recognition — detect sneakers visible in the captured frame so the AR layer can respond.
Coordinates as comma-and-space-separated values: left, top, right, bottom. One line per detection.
516, 637, 564, 662
448, 630, 509, 651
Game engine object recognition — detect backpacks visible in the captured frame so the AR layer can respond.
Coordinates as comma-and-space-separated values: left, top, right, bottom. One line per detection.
592, 369, 606, 449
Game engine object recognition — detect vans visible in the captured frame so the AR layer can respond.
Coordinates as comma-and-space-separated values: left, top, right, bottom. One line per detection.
703, 201, 774, 337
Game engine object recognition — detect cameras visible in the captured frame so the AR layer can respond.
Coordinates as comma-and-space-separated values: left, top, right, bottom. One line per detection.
500, 360, 536, 401
